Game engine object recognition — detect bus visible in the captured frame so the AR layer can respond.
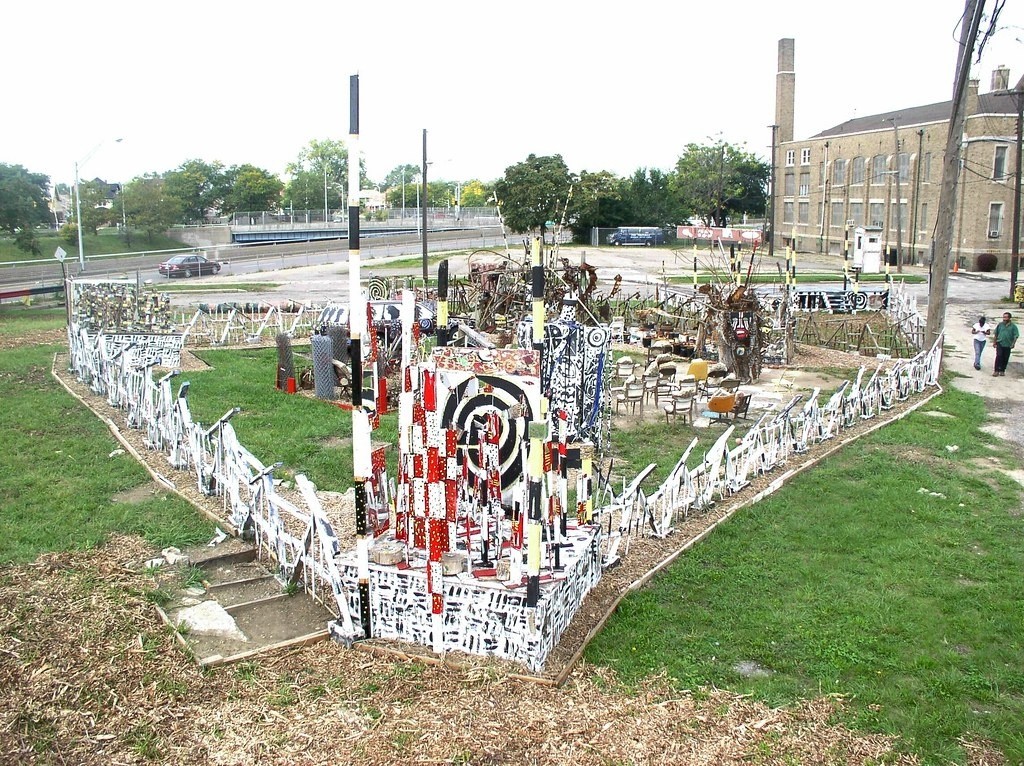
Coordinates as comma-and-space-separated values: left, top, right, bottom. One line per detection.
605, 227, 665, 247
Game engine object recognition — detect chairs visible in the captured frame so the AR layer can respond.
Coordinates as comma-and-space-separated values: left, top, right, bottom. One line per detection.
600, 316, 752, 430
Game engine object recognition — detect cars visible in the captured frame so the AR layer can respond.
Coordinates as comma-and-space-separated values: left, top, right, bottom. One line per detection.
158, 254, 221, 278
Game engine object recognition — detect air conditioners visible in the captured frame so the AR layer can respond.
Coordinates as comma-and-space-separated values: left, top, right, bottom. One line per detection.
847, 220, 854, 226
990, 231, 998, 237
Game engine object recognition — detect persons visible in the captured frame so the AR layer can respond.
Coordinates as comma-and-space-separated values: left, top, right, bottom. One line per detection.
971, 317, 990, 370
992, 312, 1019, 377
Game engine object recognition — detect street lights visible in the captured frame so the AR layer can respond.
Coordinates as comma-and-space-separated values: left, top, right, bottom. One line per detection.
335, 182, 344, 223
448, 183, 457, 222
416, 160, 433, 241
73, 136, 124, 270
325, 162, 330, 222
373, 185, 381, 210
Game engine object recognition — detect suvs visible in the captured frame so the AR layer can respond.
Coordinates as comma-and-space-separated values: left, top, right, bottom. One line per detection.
311, 300, 472, 357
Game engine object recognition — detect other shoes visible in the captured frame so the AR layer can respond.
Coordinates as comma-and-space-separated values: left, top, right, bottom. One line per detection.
1000, 372, 1004, 376
974, 364, 980, 370
993, 372, 999, 376
